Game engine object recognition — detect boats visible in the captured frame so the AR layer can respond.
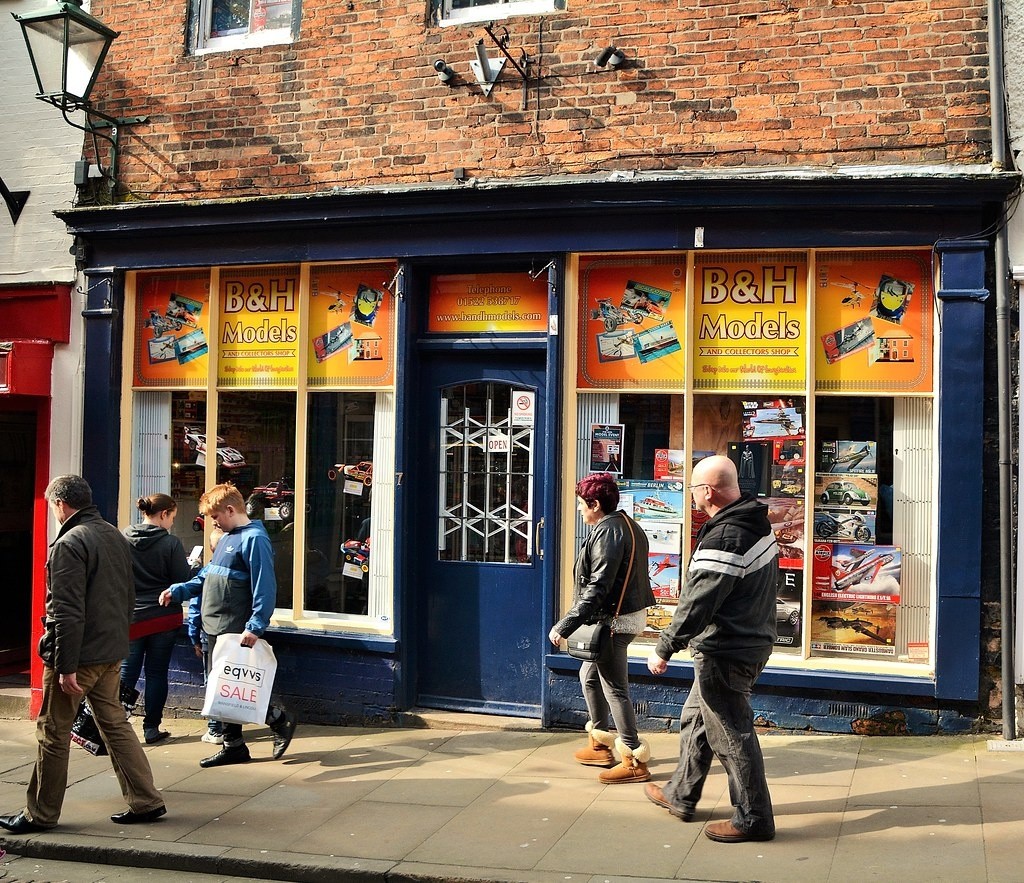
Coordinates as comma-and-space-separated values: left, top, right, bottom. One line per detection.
633, 486, 679, 518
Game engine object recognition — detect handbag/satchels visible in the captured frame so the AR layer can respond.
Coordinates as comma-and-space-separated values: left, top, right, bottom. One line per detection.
200, 633, 277, 726
566, 623, 613, 661
70, 680, 140, 757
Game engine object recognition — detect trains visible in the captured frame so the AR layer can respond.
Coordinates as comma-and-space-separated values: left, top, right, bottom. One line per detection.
355, 286, 381, 323
875, 278, 910, 320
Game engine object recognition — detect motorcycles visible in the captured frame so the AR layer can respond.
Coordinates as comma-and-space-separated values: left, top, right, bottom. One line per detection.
814, 510, 871, 542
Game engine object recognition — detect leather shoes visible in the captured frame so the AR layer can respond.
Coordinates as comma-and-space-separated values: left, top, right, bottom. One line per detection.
644, 783, 693, 822
200, 742, 251, 768
0, 810, 54, 833
111, 803, 167, 824
269, 710, 297, 760
705, 819, 756, 843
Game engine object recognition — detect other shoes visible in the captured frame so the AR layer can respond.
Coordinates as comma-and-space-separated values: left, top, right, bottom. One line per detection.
145, 732, 170, 744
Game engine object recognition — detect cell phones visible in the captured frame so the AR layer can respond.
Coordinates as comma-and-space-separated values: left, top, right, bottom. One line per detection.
187, 545, 204, 566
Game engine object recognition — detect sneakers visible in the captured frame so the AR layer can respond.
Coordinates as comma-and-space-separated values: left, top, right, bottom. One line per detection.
201, 731, 224, 744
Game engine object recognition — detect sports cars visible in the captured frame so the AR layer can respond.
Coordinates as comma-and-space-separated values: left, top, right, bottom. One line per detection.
183, 424, 247, 470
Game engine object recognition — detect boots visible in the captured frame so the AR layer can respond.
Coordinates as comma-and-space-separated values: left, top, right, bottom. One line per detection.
573, 722, 616, 765
598, 736, 652, 784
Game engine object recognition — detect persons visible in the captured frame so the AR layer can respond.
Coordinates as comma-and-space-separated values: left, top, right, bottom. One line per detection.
645, 454, 782, 844
119, 493, 203, 745
186, 526, 225, 743
547, 473, 657, 784
0, 475, 165, 834
278, 488, 371, 615
157, 482, 299, 768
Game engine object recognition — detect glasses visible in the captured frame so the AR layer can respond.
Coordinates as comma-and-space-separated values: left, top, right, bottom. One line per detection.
687, 482, 716, 493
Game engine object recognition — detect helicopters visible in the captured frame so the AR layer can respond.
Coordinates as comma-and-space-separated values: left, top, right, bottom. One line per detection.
321, 285, 350, 316
830, 275, 874, 309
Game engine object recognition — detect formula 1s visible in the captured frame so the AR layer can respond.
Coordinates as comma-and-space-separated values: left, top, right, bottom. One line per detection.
590, 297, 644, 333
143, 307, 183, 339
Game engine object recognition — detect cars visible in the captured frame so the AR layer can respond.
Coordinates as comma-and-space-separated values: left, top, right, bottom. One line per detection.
820, 480, 871, 507
192, 514, 204, 532
776, 597, 800, 626
327, 460, 373, 487
340, 537, 370, 573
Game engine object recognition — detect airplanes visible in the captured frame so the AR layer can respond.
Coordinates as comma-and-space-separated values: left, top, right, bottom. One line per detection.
651, 555, 678, 577
754, 409, 798, 435
828, 553, 896, 593
770, 505, 804, 541
160, 341, 174, 352
835, 548, 876, 571
835, 442, 873, 471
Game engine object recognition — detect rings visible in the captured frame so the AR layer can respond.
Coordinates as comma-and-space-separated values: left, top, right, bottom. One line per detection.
554, 635, 557, 638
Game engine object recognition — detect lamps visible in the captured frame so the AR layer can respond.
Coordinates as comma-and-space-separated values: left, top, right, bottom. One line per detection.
10, 0, 149, 206
594, 45, 625, 66
434, 58, 453, 82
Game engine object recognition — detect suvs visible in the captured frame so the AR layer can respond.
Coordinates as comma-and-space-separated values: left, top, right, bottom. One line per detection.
243, 474, 316, 522
